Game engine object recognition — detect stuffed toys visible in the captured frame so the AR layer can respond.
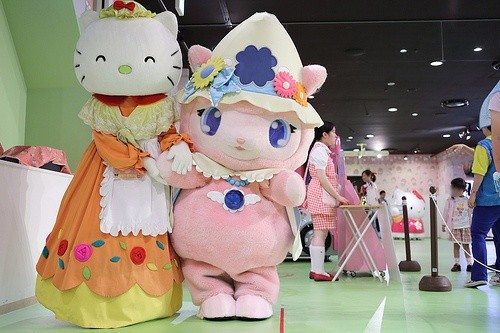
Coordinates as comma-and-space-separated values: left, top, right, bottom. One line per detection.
156, 12, 327, 321
36, 0, 192, 329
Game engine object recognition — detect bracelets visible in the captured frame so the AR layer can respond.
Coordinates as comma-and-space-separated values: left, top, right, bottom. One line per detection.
470, 189, 477, 195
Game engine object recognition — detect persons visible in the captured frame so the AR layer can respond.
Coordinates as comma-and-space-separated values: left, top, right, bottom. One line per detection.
378, 190, 388, 206
362, 170, 380, 228
463, 125, 500, 289
306, 121, 351, 282
441, 178, 473, 272
479, 80, 500, 197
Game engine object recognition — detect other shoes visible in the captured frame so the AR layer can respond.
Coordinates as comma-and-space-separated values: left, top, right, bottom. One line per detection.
309, 271, 332, 279
466, 265, 472, 272
451, 263, 461, 272
489, 274, 500, 285
314, 273, 339, 281
464, 280, 487, 288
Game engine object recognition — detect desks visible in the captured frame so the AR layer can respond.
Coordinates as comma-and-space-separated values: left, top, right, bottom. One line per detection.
0, 158, 74, 315
332, 205, 386, 283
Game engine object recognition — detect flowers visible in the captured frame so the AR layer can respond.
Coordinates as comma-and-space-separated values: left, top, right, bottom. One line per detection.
191, 57, 309, 107
